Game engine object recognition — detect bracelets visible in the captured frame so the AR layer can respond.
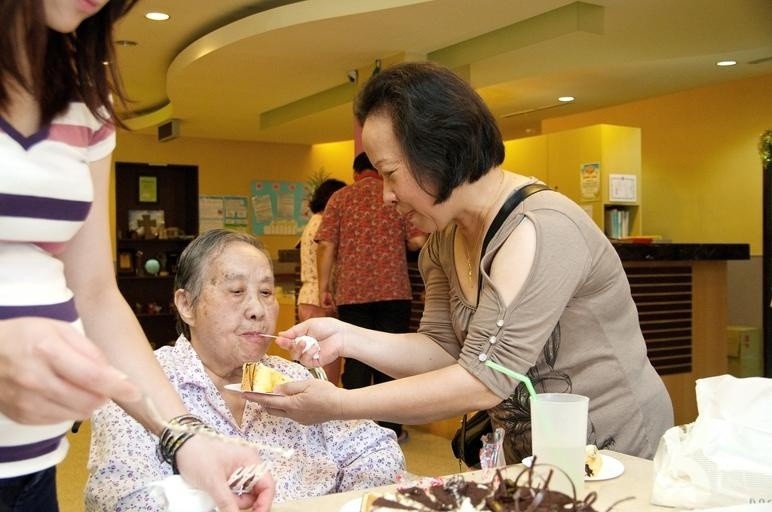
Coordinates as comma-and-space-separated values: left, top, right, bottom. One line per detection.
154, 413, 206, 476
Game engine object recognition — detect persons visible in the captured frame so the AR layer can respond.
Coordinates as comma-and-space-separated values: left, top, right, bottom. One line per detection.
241, 62, 675, 464
297, 152, 429, 444
84, 229, 407, 512
1, 0, 275, 511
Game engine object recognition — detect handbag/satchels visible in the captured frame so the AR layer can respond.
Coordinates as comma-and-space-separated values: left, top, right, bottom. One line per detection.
451, 410, 493, 467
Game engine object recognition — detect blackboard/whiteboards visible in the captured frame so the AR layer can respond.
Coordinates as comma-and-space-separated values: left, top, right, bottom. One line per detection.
198, 195, 249, 236
251, 180, 312, 236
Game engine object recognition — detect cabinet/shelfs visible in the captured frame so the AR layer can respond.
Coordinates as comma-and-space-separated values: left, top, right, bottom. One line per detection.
114, 162, 198, 351
500, 125, 642, 240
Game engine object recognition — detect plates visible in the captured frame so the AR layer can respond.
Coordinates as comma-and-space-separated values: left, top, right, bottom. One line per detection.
524, 454, 625, 482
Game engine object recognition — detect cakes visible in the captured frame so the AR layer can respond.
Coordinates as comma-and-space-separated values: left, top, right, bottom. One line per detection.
586, 444, 602, 478
359, 457, 635, 511
242, 358, 288, 395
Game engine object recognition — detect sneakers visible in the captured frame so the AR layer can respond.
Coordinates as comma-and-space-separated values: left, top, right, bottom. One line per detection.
398, 430, 409, 442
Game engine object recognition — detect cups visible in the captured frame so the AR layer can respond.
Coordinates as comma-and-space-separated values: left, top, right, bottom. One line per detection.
529, 392, 589, 500
